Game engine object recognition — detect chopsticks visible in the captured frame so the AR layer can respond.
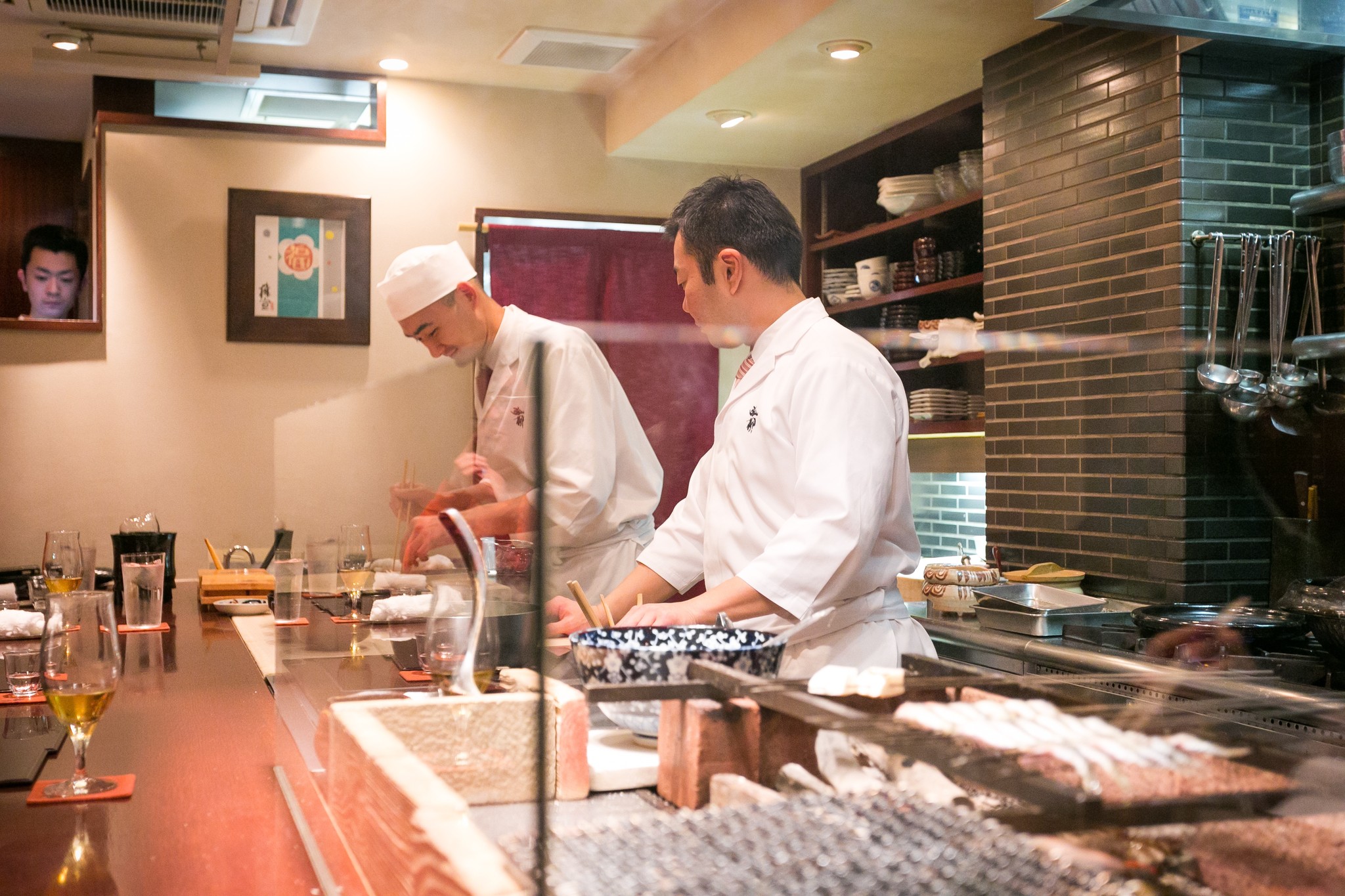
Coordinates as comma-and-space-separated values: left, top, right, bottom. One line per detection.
389, 458, 419, 573
565, 579, 604, 631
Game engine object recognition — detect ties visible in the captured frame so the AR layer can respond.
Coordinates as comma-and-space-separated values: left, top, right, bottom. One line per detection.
734, 354, 754, 389
475, 367, 493, 408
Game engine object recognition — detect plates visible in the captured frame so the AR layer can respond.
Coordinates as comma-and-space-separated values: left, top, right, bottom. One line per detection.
908, 388, 984, 421
212, 598, 270, 616
1133, 600, 1306, 637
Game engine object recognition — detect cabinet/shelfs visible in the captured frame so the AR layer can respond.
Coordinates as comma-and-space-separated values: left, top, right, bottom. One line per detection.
799, 87, 986, 473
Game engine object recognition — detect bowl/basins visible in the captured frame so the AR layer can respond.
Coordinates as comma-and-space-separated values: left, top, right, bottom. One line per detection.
879, 303, 944, 362
569, 623, 786, 749
921, 562, 1000, 616
821, 236, 984, 307
874, 148, 985, 218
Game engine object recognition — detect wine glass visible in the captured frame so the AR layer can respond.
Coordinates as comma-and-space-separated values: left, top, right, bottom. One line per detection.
39, 590, 123, 795
339, 523, 371, 620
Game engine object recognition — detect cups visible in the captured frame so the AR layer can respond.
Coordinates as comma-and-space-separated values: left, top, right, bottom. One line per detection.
121, 551, 162, 629
271, 548, 303, 620
424, 574, 501, 696
0, 598, 17, 611
42, 528, 83, 593
29, 575, 46, 612
2, 650, 42, 698
306, 533, 338, 598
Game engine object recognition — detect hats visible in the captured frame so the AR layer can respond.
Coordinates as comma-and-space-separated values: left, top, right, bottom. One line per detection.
376, 241, 478, 322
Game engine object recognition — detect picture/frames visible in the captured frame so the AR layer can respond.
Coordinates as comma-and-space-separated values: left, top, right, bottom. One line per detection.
226, 187, 371, 345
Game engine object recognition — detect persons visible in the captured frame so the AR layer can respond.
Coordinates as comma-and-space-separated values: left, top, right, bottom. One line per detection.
377, 241, 666, 610
541, 167, 940, 681
16, 223, 90, 321
